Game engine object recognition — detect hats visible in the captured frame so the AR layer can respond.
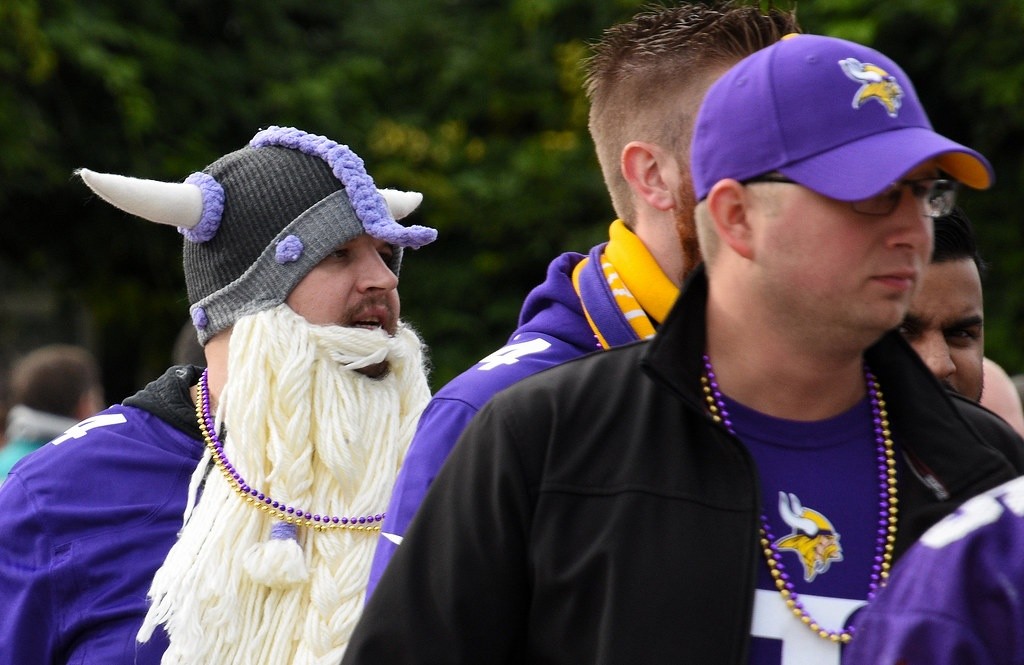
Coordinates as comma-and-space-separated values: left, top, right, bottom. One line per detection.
689, 33, 992, 208
78, 126, 438, 347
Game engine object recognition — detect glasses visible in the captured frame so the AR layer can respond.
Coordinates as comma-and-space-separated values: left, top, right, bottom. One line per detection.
741, 175, 957, 218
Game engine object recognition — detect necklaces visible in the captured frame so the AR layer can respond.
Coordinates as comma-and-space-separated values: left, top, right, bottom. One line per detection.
699, 354, 900, 646
195, 365, 388, 535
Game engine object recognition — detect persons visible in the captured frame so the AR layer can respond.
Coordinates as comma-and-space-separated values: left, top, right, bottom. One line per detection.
0, 347, 107, 486
0, 128, 435, 664
975, 355, 1024, 440
838, 465, 1024, 665
362, 0, 805, 604
895, 212, 987, 409
336, 30, 1023, 664
